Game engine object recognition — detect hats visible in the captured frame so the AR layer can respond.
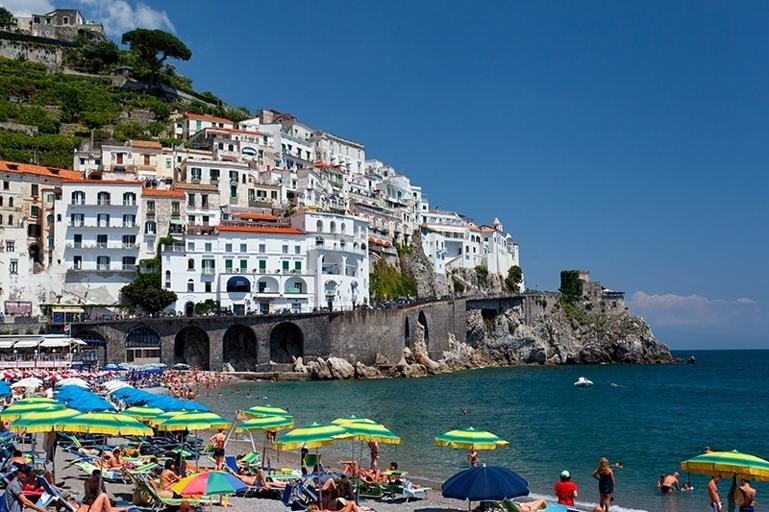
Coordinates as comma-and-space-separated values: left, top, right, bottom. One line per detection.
561, 470, 570, 478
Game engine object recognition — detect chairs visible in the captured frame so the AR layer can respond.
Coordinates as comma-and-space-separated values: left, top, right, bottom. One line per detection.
0, 429, 580, 512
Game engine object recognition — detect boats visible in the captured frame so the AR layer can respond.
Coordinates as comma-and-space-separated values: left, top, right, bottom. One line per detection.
575, 376, 594, 388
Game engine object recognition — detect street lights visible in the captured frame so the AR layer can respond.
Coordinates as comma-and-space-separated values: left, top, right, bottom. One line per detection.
52, 349, 56, 367
14, 350, 18, 367
34, 350, 37, 366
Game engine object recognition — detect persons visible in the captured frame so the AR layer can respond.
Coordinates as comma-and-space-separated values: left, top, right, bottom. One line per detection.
354, 293, 415, 311
554, 470, 577, 508
337, 441, 421, 489
467, 448, 479, 466
656, 472, 694, 494
197, 310, 218, 317
73, 312, 91, 320
232, 466, 289, 489
94, 311, 184, 320
592, 458, 625, 511
704, 446, 711, 453
305, 474, 369, 511
514, 499, 548, 511
270, 307, 291, 315
1, 349, 243, 512
312, 305, 331, 314
247, 306, 258, 315
708, 474, 756, 512
264, 428, 278, 441
